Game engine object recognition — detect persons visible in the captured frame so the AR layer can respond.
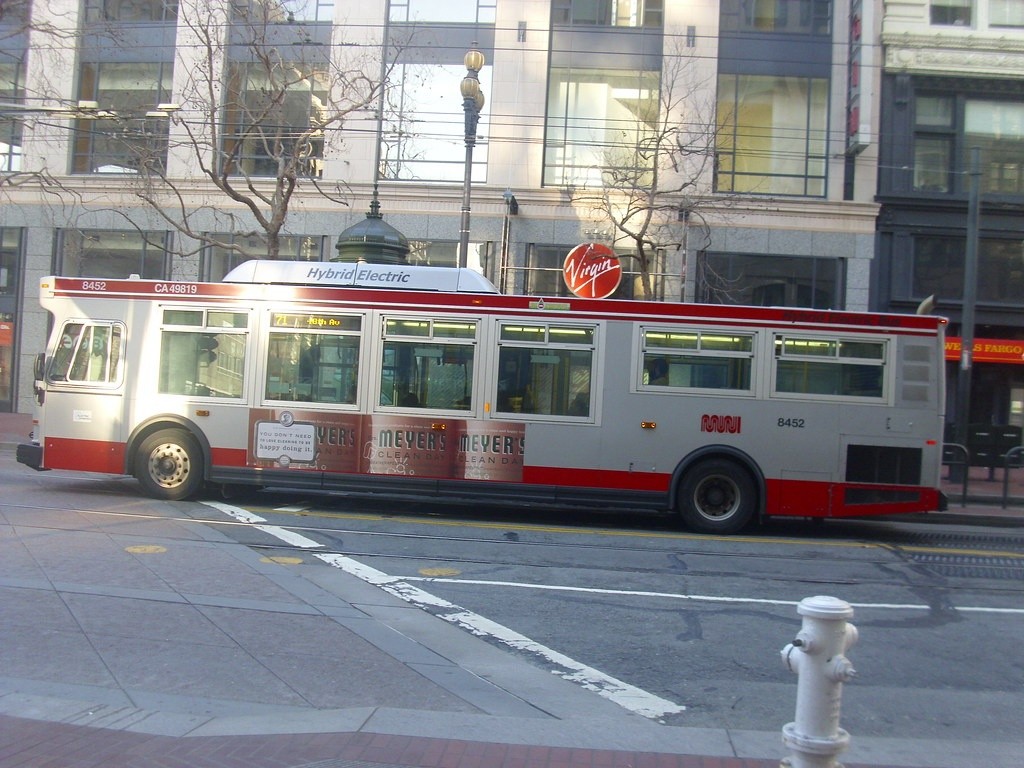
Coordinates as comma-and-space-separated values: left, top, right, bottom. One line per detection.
648, 358, 668, 386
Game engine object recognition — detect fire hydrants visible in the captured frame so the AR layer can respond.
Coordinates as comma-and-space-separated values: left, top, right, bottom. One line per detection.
773, 593, 863, 768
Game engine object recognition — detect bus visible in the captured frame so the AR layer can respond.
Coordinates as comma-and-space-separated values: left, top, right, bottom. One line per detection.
10, 257, 954, 536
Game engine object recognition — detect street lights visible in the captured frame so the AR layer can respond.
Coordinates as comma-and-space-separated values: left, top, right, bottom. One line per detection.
457, 48, 488, 269
